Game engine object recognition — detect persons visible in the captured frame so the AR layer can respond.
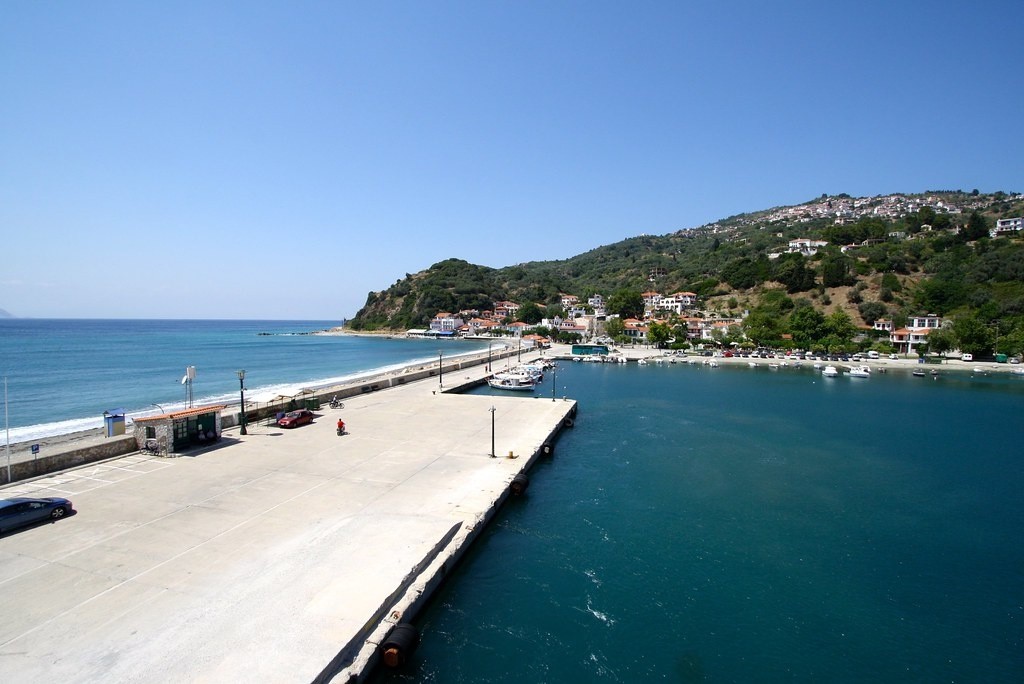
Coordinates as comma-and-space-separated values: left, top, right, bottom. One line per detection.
337, 419, 344, 432
485, 365, 488, 372
333, 395, 338, 407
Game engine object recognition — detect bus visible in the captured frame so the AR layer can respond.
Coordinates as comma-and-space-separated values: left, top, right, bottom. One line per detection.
571, 345, 609, 355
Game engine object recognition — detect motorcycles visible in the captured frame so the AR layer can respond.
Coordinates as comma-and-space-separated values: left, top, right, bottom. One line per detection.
329, 401, 345, 409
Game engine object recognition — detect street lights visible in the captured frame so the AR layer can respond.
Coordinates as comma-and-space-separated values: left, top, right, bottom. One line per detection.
235, 370, 248, 435
489, 406, 496, 457
489, 340, 494, 372
438, 350, 443, 388
552, 367, 555, 402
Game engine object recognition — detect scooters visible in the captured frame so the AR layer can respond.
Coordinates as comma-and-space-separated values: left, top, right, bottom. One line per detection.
337, 424, 345, 436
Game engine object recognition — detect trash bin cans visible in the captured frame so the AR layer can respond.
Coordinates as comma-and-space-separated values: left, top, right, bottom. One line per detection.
304, 397, 320, 410
102, 407, 128, 438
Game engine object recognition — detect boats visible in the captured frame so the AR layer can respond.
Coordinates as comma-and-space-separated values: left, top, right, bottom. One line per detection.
913, 368, 926, 375
573, 353, 887, 378
488, 359, 555, 390
929, 369, 939, 380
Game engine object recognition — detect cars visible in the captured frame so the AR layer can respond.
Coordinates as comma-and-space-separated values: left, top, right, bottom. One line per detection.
278, 409, 314, 428
663, 351, 860, 362
1011, 357, 1020, 363
918, 356, 929, 364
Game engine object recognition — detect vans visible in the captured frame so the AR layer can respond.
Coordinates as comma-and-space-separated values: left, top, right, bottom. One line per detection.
961, 354, 972, 360
891, 354, 898, 360
0, 496, 72, 536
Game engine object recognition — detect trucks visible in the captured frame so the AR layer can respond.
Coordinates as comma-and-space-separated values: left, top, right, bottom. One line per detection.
869, 351, 879, 359
